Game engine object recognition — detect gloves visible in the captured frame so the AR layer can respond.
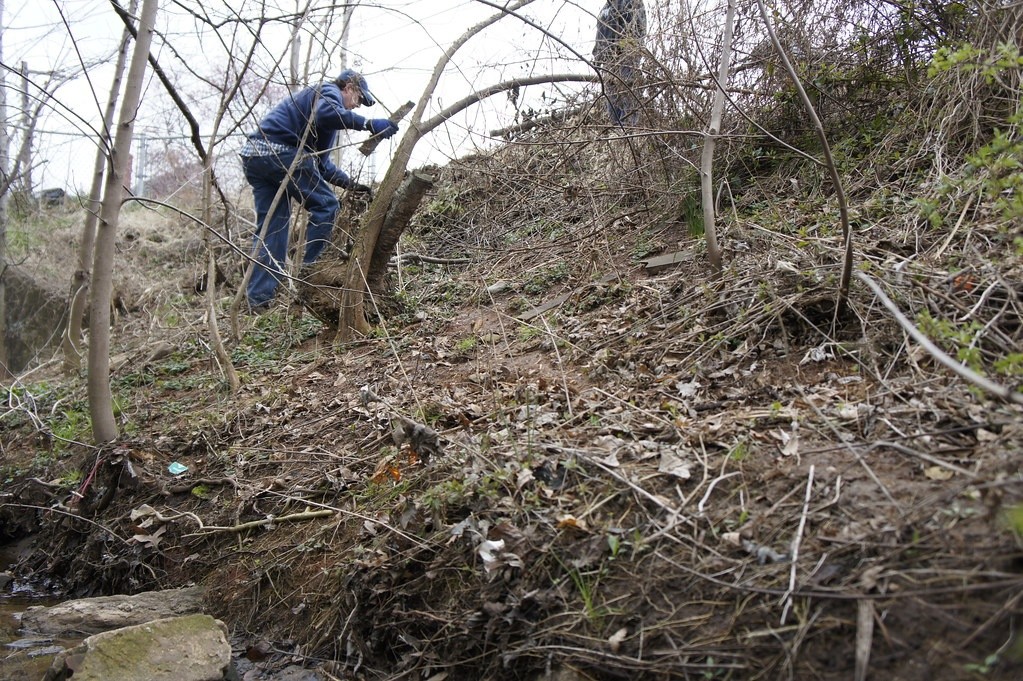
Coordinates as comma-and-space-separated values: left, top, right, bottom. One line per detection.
352, 183, 373, 205
366, 119, 399, 139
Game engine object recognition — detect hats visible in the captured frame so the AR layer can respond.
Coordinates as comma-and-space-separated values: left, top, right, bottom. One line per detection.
338, 69, 376, 106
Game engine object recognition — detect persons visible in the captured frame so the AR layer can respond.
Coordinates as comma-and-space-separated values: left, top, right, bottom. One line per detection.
593, 0, 646, 126
238, 68, 399, 308
